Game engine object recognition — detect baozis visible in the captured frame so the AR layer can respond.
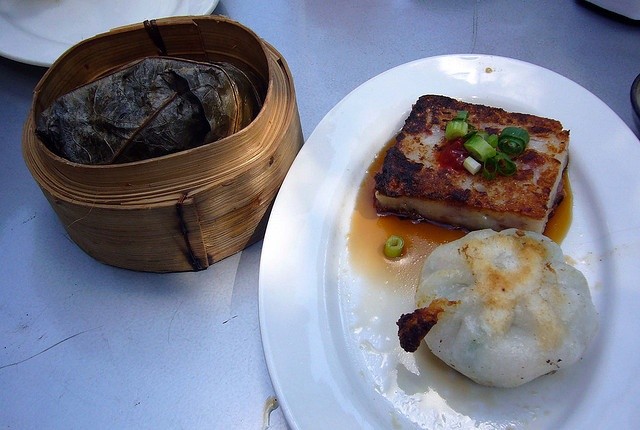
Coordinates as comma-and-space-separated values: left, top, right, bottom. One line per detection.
415, 230, 600, 390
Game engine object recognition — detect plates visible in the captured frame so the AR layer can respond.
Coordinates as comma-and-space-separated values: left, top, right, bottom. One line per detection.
0, 0, 220, 73
259, 54, 640, 430
